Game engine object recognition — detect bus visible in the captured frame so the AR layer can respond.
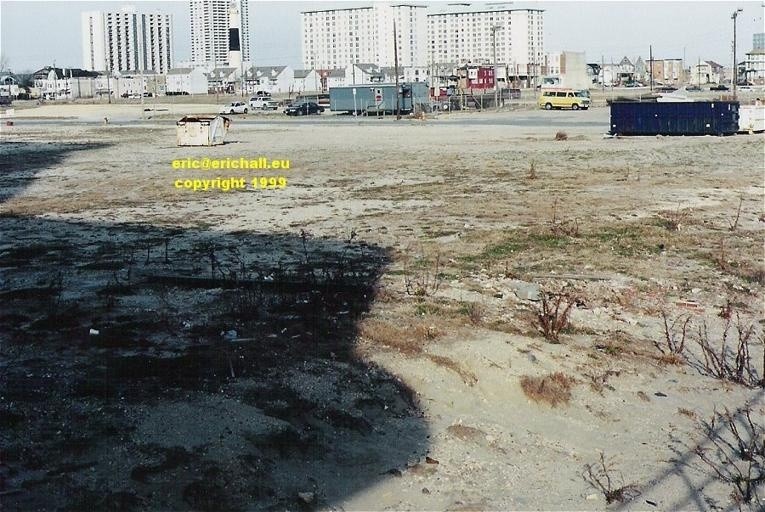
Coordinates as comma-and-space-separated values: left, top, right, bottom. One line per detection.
538, 88, 591, 111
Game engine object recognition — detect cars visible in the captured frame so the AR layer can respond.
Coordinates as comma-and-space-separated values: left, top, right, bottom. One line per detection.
624, 80, 643, 87
732, 80, 765, 93
219, 100, 249, 115
282, 100, 325, 116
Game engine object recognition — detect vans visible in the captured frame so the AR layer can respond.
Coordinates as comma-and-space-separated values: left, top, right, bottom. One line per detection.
600, 79, 618, 88
248, 96, 279, 110
497, 88, 521, 100
256, 90, 272, 96
